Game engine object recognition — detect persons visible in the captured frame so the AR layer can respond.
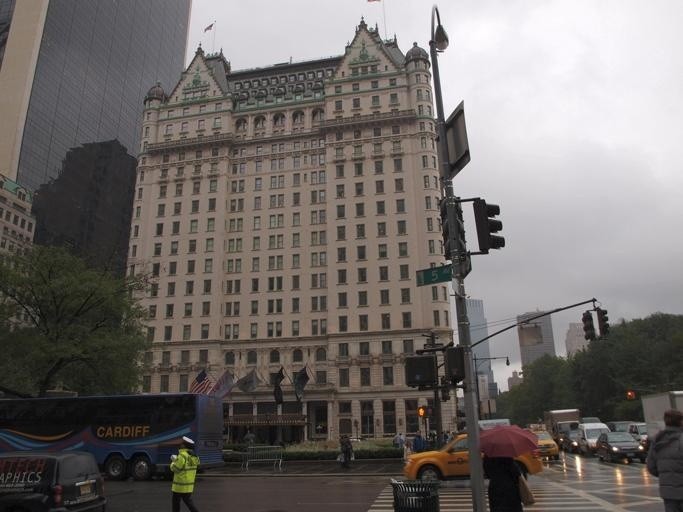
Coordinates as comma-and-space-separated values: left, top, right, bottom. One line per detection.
482, 453, 524, 512
393, 433, 400, 448
170, 436, 201, 511
339, 435, 345, 454
412, 430, 427, 452
514, 460, 527, 482
428, 430, 453, 444
399, 433, 406, 448
646, 409, 683, 511
343, 436, 353, 468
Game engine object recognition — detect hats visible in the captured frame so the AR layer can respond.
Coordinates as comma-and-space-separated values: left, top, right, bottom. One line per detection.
182, 436, 195, 445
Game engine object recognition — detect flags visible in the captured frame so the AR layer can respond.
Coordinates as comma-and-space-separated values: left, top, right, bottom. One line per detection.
235, 367, 263, 392
204, 22, 213, 32
294, 364, 311, 394
208, 370, 235, 398
368, 0, 380, 2
187, 370, 212, 394
272, 367, 285, 387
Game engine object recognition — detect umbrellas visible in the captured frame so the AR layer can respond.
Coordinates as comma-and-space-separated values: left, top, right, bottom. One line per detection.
481, 423, 539, 457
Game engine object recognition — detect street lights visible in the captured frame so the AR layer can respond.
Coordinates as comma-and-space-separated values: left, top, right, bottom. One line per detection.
473, 353, 510, 419
430, 6, 487, 511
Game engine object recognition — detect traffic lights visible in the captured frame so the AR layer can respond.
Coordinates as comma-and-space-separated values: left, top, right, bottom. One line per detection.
440, 378, 452, 401
417, 407, 433, 418
582, 312, 596, 340
627, 391, 636, 401
473, 198, 504, 251
598, 308, 610, 335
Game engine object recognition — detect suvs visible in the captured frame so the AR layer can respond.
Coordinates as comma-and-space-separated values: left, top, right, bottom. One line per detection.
0, 452, 105, 510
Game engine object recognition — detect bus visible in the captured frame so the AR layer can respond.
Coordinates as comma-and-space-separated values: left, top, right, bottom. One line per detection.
1, 392, 226, 480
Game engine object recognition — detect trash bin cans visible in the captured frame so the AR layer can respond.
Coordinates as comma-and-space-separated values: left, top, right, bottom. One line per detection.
390, 480, 442, 512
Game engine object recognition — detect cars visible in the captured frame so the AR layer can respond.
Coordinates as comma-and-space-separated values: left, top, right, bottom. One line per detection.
535, 409, 648, 465
404, 433, 543, 484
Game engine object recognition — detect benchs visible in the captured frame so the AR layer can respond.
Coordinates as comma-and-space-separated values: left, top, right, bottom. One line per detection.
240, 445, 285, 473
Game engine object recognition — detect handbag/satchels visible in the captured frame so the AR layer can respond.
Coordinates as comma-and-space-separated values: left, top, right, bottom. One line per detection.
518, 468, 537, 505
